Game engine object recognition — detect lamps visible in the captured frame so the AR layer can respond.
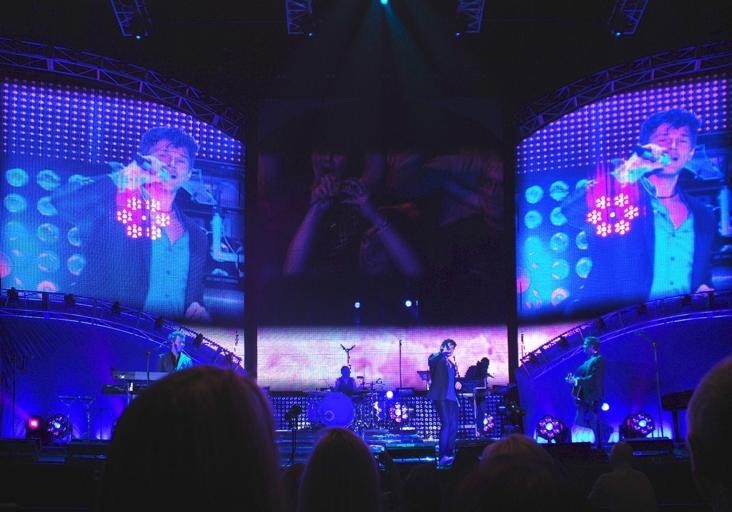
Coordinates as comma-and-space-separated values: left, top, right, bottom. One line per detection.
620, 412, 655, 439
48, 413, 73, 440
537, 416, 561, 442
24, 413, 45, 442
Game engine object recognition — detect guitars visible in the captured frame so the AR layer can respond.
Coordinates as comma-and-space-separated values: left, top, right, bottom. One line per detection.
567, 372, 584, 405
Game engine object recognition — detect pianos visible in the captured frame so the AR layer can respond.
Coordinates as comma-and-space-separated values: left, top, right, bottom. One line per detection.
113, 370, 168, 381
102, 384, 146, 394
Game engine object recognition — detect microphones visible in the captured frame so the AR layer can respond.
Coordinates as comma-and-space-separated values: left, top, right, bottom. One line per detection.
631, 144, 671, 166
652, 340, 656, 349
445, 342, 452, 354
164, 341, 172, 346
130, 152, 170, 182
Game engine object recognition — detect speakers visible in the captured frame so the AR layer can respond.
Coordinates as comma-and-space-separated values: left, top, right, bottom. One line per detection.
384, 441, 437, 469
540, 442, 590, 469
622, 436, 681, 470
0, 438, 41, 464
454, 440, 495, 469
69, 438, 112, 464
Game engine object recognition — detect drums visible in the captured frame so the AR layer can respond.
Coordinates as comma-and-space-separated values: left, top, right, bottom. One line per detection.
319, 392, 356, 430
308, 404, 320, 422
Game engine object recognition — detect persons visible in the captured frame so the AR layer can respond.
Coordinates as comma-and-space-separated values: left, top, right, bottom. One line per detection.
279, 119, 426, 294
159, 332, 191, 372
427, 339, 462, 465
465, 357, 490, 437
565, 337, 605, 448
49, 127, 214, 325
561, 106, 715, 314
106, 360, 731, 511
